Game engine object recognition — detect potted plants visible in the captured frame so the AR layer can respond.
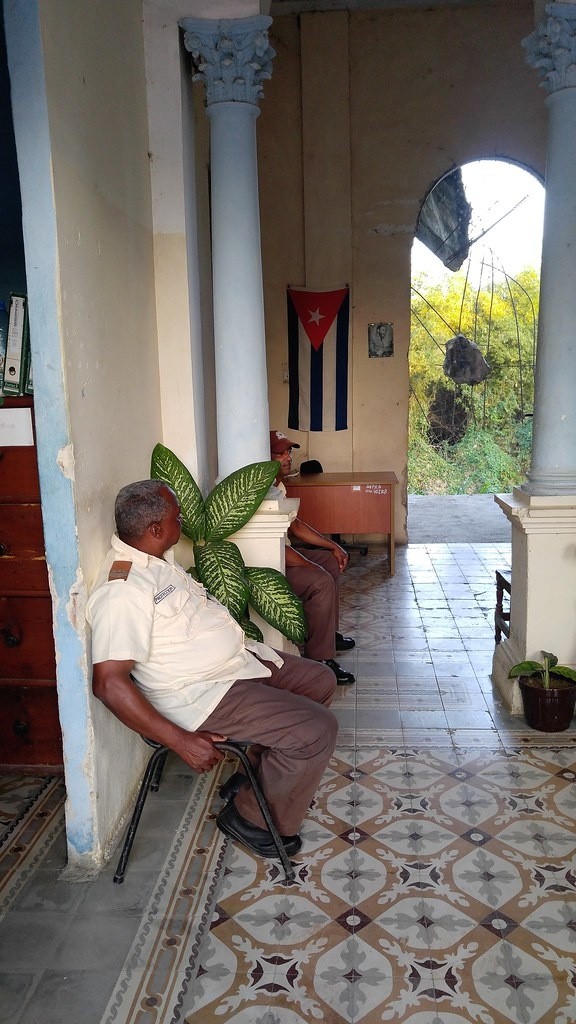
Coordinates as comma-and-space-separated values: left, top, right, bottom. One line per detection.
507, 648, 576, 733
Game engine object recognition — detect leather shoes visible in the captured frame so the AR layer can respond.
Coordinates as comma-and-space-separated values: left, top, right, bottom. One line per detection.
314, 659, 356, 684
334, 631, 355, 651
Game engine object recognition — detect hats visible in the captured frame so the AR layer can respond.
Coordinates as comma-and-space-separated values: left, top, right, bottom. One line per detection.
270, 430, 300, 453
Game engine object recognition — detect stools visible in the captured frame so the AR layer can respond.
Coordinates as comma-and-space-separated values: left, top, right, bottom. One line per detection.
494, 568, 512, 646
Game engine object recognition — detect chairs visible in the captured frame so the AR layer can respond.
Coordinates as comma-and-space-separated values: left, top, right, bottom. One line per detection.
112, 667, 296, 886
299, 459, 369, 562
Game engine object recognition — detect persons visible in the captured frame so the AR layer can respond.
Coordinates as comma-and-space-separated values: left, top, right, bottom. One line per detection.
270, 430, 356, 685
85, 479, 338, 858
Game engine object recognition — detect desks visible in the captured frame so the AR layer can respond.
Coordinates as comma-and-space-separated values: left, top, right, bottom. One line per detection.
280, 469, 399, 576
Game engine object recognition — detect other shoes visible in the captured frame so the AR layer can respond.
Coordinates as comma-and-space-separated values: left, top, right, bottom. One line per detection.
216, 794, 302, 857
217, 771, 250, 801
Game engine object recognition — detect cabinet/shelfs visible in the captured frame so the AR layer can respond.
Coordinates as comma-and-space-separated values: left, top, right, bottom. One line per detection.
0, 445, 64, 778
